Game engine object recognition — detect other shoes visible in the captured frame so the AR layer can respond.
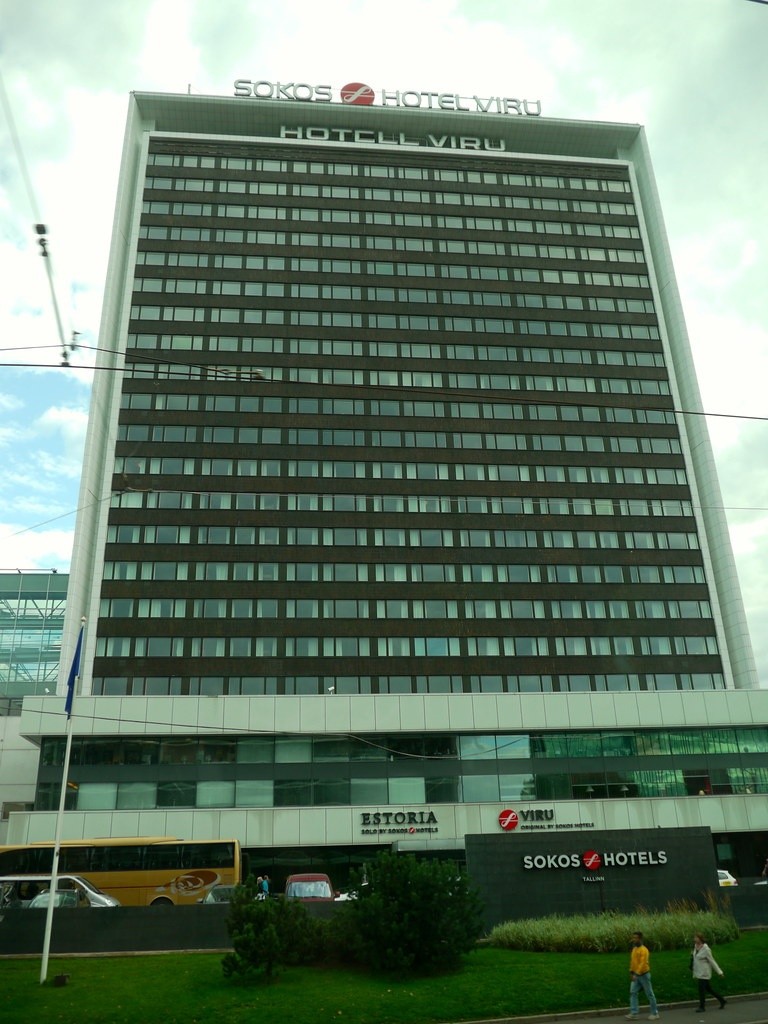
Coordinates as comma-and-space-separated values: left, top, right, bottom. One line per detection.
719, 1000, 726, 1009
696, 1008, 706, 1012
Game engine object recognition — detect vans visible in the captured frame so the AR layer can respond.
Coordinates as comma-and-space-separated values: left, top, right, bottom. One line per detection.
284, 873, 341, 902
0, 874, 123, 908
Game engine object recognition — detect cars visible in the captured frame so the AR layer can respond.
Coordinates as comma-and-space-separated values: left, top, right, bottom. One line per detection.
196, 883, 246, 904
716, 870, 739, 887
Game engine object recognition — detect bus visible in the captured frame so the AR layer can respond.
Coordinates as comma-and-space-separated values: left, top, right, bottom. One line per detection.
0, 837, 242, 906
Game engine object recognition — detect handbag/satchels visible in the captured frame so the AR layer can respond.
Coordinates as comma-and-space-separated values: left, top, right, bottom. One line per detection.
689, 944, 695, 970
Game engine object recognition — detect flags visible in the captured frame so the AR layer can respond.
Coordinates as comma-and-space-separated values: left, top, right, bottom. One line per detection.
64, 627, 82, 720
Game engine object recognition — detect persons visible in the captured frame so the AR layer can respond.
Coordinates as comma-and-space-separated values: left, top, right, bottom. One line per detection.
626, 931, 661, 1020
254, 874, 269, 900
690, 933, 727, 1013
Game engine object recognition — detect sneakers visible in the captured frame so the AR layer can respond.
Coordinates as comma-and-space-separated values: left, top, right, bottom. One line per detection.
625, 1014, 639, 1019
647, 1014, 659, 1020
